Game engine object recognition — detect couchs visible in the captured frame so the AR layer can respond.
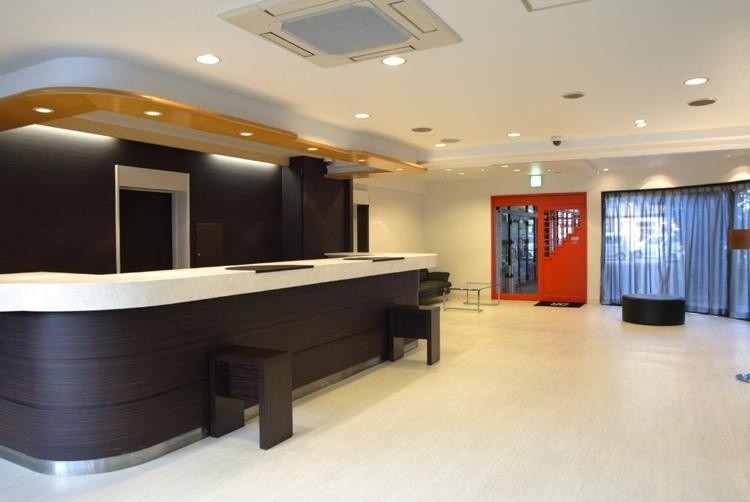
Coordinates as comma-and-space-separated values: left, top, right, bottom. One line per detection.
420, 268, 452, 306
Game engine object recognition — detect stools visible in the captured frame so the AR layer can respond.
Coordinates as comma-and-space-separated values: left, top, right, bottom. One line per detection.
621, 294, 688, 327
384, 302, 442, 365
207, 342, 293, 450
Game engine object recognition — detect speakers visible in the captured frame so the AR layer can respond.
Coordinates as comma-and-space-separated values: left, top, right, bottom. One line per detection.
288, 156, 328, 180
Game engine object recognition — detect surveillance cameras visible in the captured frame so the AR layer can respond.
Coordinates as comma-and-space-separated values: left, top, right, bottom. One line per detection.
551, 136, 562, 146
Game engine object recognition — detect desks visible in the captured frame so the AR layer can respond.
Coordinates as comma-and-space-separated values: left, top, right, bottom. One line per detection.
443, 280, 501, 314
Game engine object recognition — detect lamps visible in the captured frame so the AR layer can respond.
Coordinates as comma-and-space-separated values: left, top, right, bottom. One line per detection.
529, 175, 543, 188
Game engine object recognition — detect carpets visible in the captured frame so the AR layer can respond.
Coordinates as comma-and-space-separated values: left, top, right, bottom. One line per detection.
534, 301, 584, 308
